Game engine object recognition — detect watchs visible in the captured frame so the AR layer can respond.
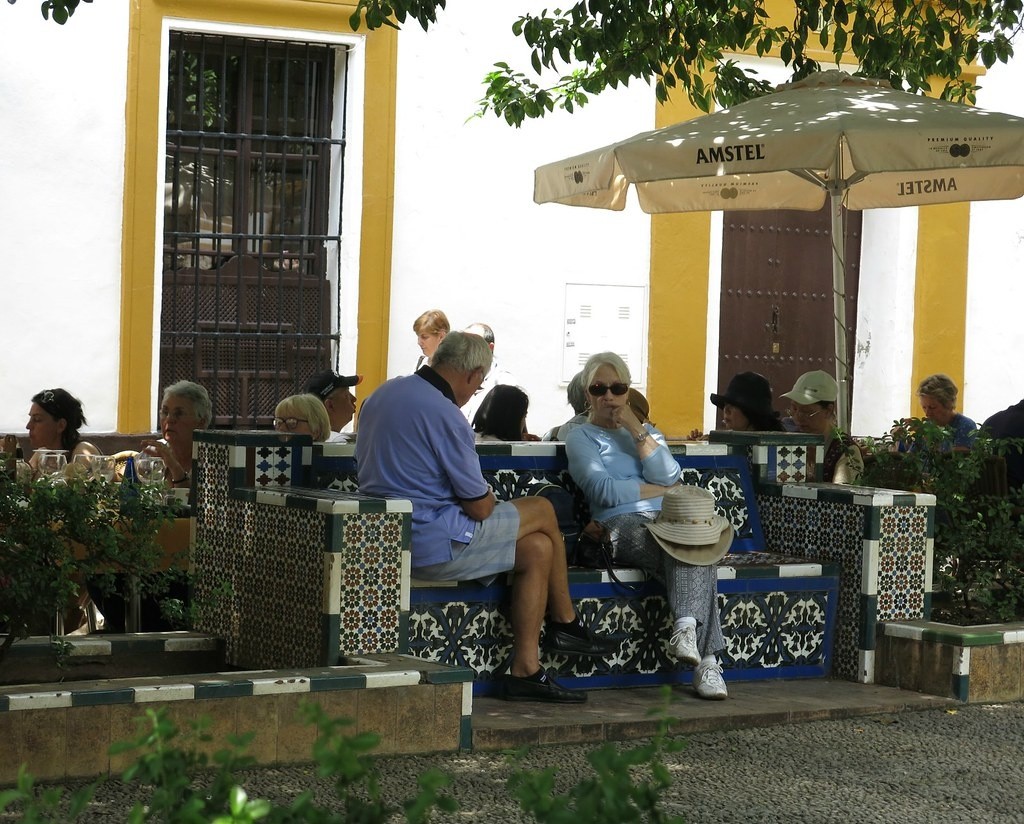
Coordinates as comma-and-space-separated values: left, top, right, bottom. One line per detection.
634, 431, 649, 444
171, 471, 190, 484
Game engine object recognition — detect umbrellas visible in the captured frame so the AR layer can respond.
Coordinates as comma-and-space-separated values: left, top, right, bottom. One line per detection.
533, 69, 1024, 439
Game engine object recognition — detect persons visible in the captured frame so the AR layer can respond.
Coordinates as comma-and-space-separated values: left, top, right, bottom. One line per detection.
471, 383, 540, 441
687, 370, 786, 475
273, 393, 331, 442
302, 368, 363, 442
78, 380, 213, 634
778, 370, 865, 486
460, 322, 518, 424
565, 352, 727, 699
413, 309, 450, 371
977, 399, 1024, 539
356, 331, 618, 703
892, 374, 978, 474
626, 387, 656, 427
0, 387, 104, 495
541, 370, 590, 441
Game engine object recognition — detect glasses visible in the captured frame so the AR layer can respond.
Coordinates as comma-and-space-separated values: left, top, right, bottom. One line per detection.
40, 390, 56, 406
272, 417, 308, 430
786, 408, 824, 420
159, 408, 197, 420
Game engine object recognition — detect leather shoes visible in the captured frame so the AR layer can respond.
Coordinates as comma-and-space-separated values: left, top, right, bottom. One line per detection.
504, 673, 589, 703
548, 627, 620, 654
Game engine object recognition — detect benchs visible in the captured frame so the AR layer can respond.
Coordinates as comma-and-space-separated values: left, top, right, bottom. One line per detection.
192, 427, 845, 681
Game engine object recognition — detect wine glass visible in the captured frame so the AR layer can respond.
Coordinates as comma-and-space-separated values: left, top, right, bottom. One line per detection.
32, 449, 51, 488
42, 455, 67, 488
51, 450, 70, 487
73, 455, 101, 493
137, 459, 166, 509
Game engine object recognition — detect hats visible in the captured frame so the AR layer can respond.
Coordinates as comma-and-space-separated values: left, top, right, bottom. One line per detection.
710, 372, 780, 417
305, 371, 359, 400
642, 486, 736, 564
780, 370, 838, 405
627, 388, 651, 423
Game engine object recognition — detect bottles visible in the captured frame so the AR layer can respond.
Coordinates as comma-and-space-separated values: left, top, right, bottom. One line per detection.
16, 448, 31, 486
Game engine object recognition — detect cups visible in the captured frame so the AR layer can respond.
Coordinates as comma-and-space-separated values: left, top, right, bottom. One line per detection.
172, 488, 190, 506
92, 456, 116, 487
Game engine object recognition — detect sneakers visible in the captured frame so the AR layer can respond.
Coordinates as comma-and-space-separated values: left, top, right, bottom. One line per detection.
692, 662, 728, 698
669, 625, 701, 667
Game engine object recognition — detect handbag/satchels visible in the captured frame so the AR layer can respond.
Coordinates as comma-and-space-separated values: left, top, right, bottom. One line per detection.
569, 518, 619, 570
525, 482, 588, 566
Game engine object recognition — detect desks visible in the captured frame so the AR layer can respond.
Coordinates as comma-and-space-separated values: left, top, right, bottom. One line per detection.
0, 510, 197, 633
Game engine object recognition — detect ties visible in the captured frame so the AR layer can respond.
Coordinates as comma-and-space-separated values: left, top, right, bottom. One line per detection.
588, 383, 630, 396
